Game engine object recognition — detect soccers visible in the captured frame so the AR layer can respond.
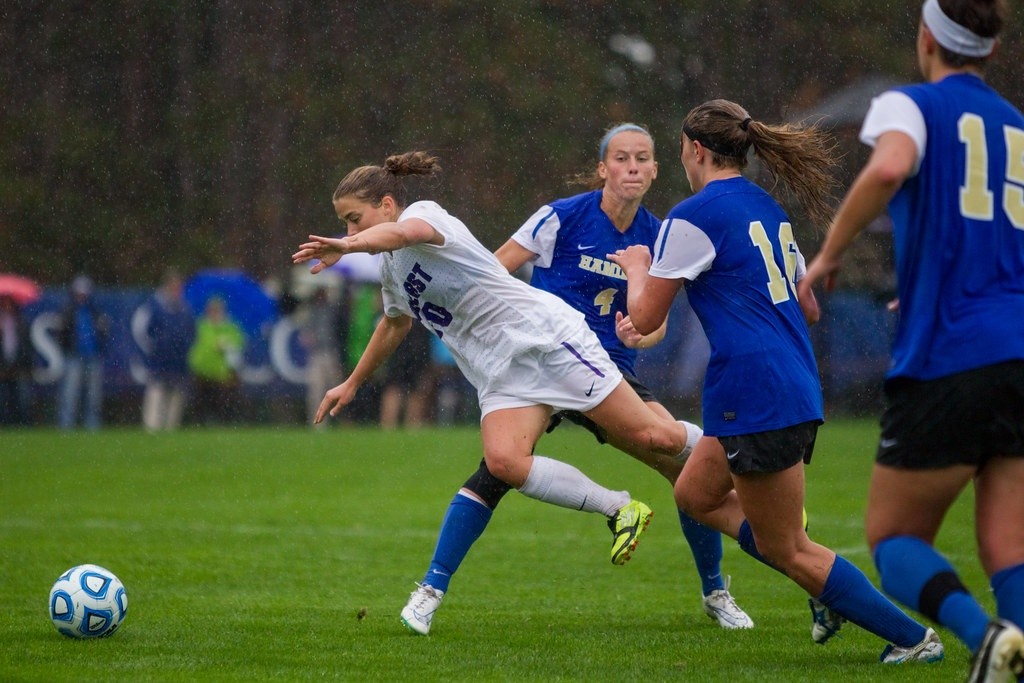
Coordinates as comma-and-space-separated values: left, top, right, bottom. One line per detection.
48, 563, 128, 639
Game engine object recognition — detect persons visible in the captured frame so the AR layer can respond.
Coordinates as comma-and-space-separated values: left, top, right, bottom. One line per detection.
0, 295, 32, 426
292, 285, 479, 428
131, 271, 195, 432
606, 99, 947, 664
289, 151, 810, 568
56, 276, 109, 429
796, 0, 1024, 683
189, 299, 246, 426
398, 124, 756, 639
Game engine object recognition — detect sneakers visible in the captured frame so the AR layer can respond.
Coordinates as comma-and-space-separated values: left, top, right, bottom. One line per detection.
809, 597, 848, 646
880, 627, 945, 664
702, 575, 754, 630
966, 620, 1024, 683
802, 506, 808, 532
399, 581, 444, 637
607, 499, 654, 566
